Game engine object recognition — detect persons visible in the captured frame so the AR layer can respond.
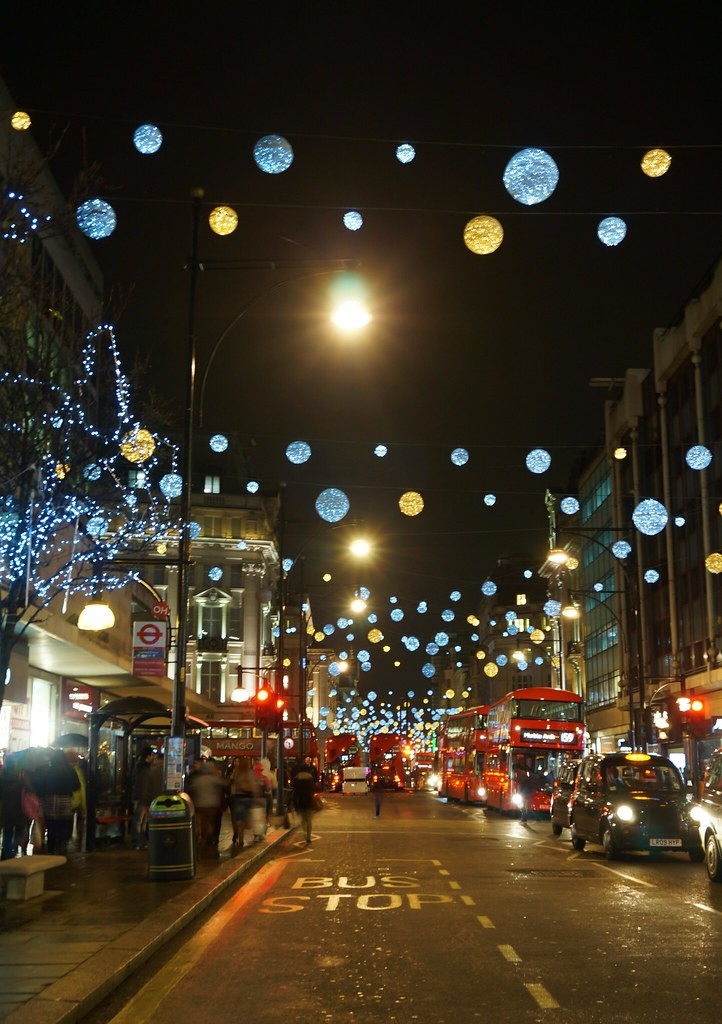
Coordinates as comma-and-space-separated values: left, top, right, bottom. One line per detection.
129, 748, 317, 857
0, 747, 86, 857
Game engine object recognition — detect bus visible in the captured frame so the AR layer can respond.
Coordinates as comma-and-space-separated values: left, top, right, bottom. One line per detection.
369, 733, 413, 791
411, 753, 436, 786
200, 720, 321, 788
437, 705, 489, 802
481, 687, 587, 811
325, 734, 361, 788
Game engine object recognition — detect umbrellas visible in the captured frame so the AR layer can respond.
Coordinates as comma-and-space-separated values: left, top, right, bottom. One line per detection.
50, 734, 88, 747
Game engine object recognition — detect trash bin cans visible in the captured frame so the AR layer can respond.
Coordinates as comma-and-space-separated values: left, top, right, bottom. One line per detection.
149, 795, 193, 880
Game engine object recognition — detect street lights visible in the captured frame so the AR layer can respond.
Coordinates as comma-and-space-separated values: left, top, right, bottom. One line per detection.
297, 582, 368, 761
76, 258, 375, 793
547, 520, 647, 753
230, 522, 376, 816
513, 621, 563, 690
563, 589, 636, 750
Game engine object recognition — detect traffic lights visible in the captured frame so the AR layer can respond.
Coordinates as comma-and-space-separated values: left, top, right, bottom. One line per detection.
257, 689, 269, 702
691, 698, 706, 739
275, 697, 285, 709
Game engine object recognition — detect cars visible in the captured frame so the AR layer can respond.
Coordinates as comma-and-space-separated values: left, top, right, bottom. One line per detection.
549, 759, 585, 834
697, 747, 722, 882
568, 753, 706, 862
341, 778, 369, 796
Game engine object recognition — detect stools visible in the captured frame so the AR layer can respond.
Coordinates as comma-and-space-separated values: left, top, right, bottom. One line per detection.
0, 854, 66, 901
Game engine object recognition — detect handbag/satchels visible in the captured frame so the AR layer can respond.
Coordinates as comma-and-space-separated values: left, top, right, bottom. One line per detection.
29, 821, 41, 847
312, 793, 326, 811
22, 779, 41, 818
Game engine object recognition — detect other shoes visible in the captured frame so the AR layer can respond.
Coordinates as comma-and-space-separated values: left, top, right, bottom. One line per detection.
254, 834, 263, 842
307, 838, 312, 843
